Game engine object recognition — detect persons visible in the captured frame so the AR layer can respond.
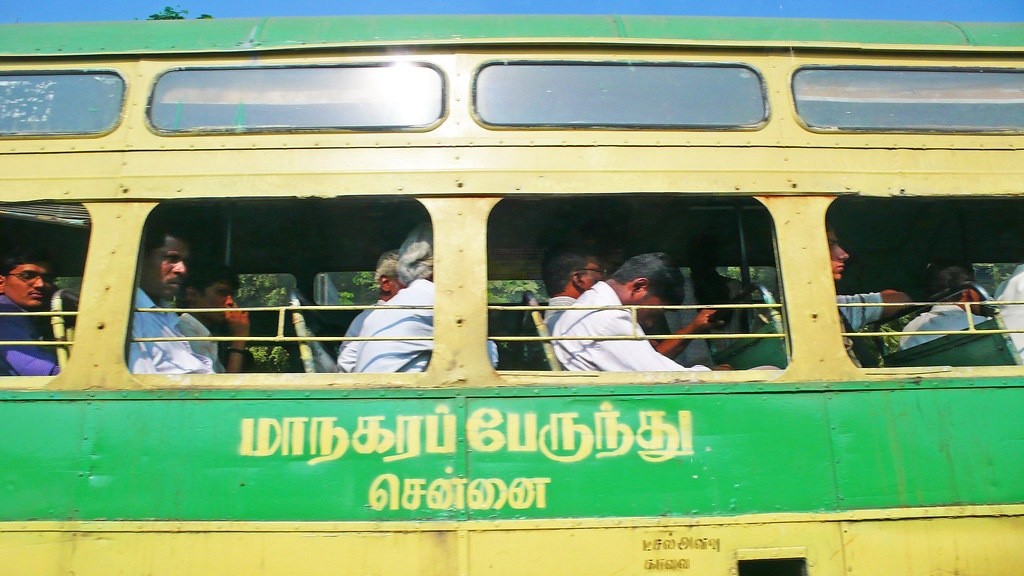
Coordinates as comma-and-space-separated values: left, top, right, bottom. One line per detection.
824, 219, 1024, 368
542, 239, 783, 371
127, 224, 256, 375
0, 252, 61, 376
337, 222, 500, 373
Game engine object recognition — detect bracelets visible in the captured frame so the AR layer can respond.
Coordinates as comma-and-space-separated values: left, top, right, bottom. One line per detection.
227, 347, 249, 355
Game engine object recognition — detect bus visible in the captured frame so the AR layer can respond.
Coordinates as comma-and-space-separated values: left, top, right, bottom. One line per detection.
0, 0, 1023, 573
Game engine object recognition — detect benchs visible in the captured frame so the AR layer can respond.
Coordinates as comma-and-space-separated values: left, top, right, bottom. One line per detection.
522, 290, 564, 371
289, 284, 335, 373
873, 280, 1023, 368
702, 282, 788, 372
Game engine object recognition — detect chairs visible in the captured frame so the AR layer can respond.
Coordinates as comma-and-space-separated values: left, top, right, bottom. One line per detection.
50, 287, 80, 373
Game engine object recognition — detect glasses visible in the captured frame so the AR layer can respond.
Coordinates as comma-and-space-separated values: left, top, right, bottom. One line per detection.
6, 269, 52, 283
568, 267, 608, 278
